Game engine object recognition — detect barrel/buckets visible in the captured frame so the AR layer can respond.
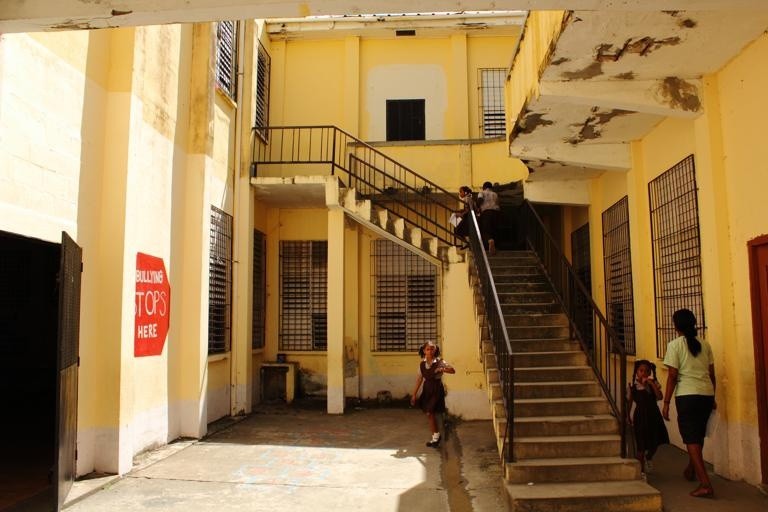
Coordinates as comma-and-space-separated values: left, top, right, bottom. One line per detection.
378, 391, 392, 404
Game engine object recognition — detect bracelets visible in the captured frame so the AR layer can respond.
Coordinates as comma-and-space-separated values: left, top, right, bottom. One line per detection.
664, 400, 670, 404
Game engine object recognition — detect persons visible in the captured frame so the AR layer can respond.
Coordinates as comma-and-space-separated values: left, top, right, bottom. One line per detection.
475, 182, 501, 257
410, 341, 456, 447
662, 309, 718, 497
454, 186, 480, 250
626, 360, 670, 480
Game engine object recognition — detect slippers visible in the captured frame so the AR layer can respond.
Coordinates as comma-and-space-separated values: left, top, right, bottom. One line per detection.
641, 460, 654, 482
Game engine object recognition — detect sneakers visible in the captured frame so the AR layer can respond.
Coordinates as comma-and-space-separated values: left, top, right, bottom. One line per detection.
489, 239, 495, 255
461, 245, 468, 250
426, 436, 441, 447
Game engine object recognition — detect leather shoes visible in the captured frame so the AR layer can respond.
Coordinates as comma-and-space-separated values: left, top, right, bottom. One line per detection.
683, 469, 713, 497
